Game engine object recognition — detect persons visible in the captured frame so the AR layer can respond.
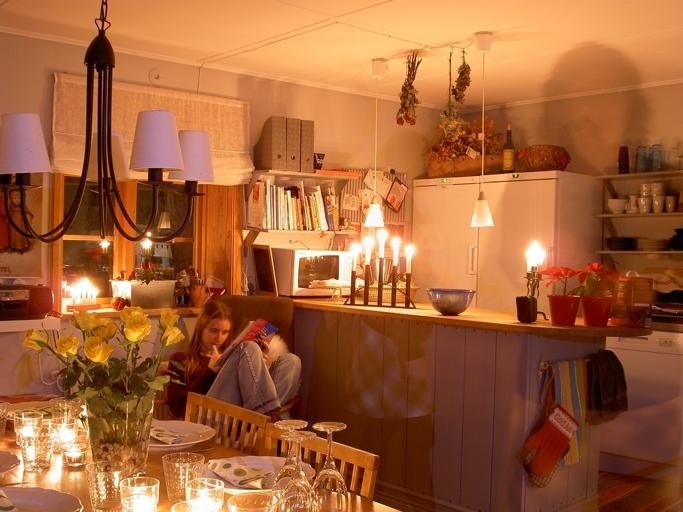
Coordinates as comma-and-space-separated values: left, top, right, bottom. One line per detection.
166, 301, 301, 455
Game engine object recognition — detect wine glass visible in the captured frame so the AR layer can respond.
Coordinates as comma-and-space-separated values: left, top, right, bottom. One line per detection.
273, 413, 349, 512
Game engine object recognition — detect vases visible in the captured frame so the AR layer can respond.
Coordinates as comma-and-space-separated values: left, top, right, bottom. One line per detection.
548, 295, 578, 327
583, 297, 612, 327
82, 397, 152, 475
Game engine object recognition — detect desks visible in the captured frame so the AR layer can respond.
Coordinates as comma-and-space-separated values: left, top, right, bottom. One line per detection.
0, 400, 400, 512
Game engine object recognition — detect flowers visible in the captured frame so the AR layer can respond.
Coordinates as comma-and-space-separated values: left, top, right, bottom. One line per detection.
121, 260, 166, 281
13, 300, 186, 439
539, 266, 581, 295
575, 258, 619, 297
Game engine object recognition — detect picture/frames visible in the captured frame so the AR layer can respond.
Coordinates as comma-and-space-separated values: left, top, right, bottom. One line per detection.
249, 242, 279, 296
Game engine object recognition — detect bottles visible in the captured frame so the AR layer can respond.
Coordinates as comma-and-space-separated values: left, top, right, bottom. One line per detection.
501, 129, 513, 173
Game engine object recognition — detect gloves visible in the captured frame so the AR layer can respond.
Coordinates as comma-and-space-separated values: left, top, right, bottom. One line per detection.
523, 404, 578, 477
522, 442, 570, 489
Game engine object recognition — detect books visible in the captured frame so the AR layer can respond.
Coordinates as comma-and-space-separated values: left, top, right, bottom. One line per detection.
196, 286, 226, 306
244, 178, 340, 231
213, 319, 276, 370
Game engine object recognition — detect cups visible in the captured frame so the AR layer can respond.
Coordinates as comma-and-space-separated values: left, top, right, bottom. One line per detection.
637, 182, 678, 214
617, 142, 663, 175
0, 401, 7, 434
625, 194, 638, 215
85, 449, 270, 511
13, 398, 88, 470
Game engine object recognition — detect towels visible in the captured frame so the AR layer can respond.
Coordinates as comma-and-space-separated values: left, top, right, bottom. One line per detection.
536, 360, 591, 466
588, 347, 627, 427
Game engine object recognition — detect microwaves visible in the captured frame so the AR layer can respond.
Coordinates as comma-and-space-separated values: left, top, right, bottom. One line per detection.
270, 247, 365, 298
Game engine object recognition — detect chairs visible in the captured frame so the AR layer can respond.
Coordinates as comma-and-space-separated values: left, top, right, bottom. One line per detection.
149, 360, 187, 420
262, 423, 378, 499
153, 296, 295, 429
180, 388, 268, 452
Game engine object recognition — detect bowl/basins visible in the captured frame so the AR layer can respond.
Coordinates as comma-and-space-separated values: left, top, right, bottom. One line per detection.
606, 199, 629, 217
426, 288, 477, 316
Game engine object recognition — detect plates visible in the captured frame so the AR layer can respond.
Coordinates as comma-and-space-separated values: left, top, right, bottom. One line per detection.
0, 451, 20, 474
145, 420, 216, 453
606, 234, 664, 250
6, 400, 67, 424
200, 454, 317, 490
0, 485, 83, 511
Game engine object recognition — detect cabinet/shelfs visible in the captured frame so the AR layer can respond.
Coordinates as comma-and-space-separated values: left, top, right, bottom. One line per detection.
410, 173, 599, 314
588, 171, 682, 334
242, 169, 357, 251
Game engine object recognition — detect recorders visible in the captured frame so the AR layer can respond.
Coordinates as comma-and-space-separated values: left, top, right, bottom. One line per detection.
0, 285, 54, 320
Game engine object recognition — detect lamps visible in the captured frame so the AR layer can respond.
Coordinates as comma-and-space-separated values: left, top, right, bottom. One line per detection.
63, 278, 103, 312
364, 48, 389, 229
0, 0, 211, 245
342, 227, 418, 309
466, 31, 496, 230
521, 232, 548, 320
